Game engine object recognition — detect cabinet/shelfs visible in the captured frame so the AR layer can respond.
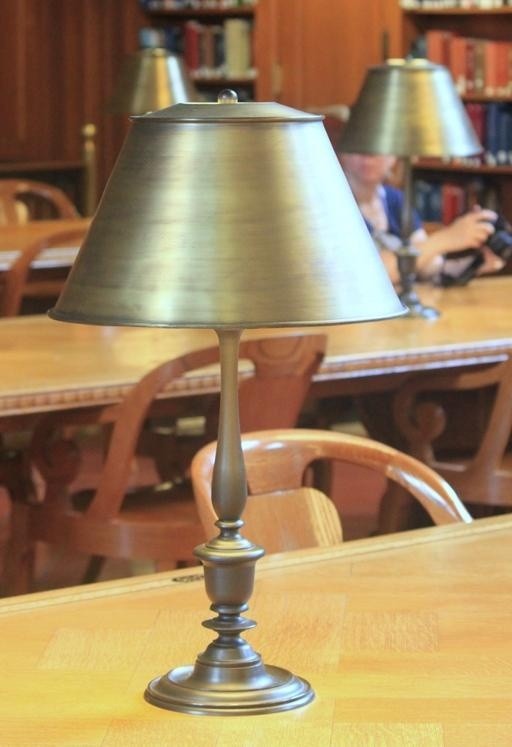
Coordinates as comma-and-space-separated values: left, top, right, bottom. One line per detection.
118, 0, 276, 101
384, 0, 511, 283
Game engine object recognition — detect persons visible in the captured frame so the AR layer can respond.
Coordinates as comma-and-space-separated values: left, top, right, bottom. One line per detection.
337, 151, 508, 285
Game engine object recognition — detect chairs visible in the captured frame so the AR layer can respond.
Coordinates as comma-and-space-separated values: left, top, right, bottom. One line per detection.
391, 356, 512, 509
41, 334, 325, 584
187, 429, 475, 545
1, 180, 511, 313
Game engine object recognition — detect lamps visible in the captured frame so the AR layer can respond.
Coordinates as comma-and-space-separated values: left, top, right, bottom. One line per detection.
103, 46, 191, 116
43, 89, 412, 712
331, 54, 485, 320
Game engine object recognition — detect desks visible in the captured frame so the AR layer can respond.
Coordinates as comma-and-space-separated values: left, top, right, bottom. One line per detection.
3, 269, 509, 420
3, 514, 512, 740
